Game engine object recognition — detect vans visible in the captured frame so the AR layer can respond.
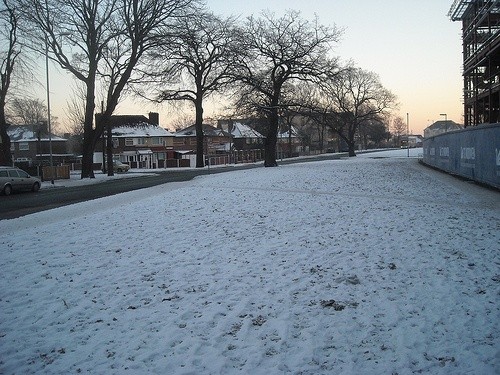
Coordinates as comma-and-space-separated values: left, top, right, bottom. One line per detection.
0, 166, 42, 196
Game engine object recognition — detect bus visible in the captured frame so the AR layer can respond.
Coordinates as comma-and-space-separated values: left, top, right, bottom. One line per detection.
401, 139, 410, 149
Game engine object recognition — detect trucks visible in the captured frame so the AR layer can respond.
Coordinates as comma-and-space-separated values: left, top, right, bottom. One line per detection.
402, 135, 424, 148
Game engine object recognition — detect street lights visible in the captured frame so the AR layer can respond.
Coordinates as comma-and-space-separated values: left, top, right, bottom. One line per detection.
440, 113, 449, 134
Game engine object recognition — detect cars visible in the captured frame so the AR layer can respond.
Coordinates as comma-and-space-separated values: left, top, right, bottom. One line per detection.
100, 159, 130, 174
